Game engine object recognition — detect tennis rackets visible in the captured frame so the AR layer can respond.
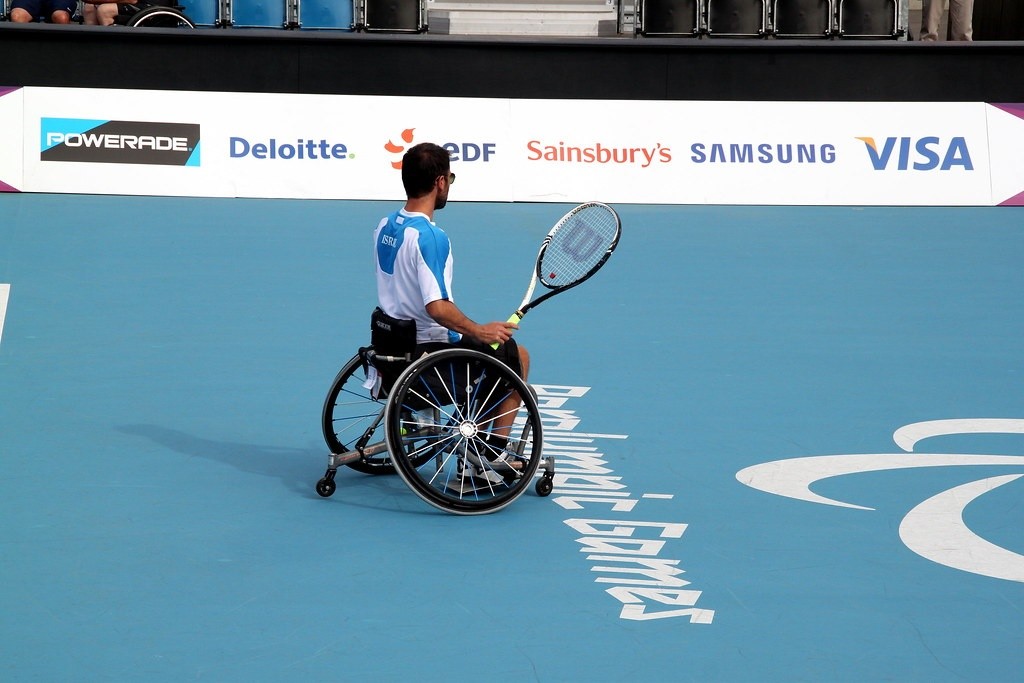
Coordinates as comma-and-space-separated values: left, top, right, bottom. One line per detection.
489, 203, 623, 350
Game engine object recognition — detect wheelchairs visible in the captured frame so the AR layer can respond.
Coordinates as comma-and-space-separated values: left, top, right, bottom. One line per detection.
315, 306, 556, 516
107, 0, 198, 28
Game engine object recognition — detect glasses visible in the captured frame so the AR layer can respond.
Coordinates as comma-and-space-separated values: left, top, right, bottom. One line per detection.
436, 173, 456, 185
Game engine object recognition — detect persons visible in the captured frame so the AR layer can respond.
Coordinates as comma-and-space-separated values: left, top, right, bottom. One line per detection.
373, 143, 530, 482
10, 0, 77, 24
918, 0, 975, 42
82, 0, 178, 28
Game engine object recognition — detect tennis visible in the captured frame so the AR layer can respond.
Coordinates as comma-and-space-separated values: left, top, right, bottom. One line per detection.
400, 427, 407, 436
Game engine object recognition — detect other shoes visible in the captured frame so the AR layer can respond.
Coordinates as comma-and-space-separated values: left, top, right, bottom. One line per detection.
475, 443, 528, 487
457, 446, 488, 484
